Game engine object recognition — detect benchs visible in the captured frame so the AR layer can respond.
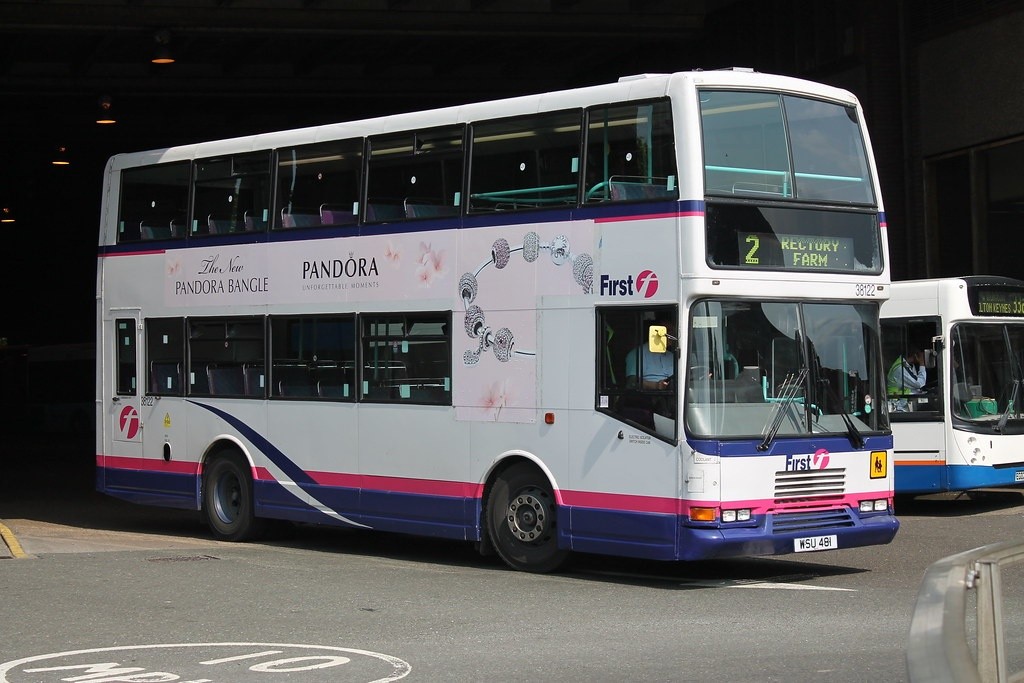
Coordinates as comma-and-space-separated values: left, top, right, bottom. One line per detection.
140, 197, 461, 240
151, 358, 453, 397
608, 174, 679, 200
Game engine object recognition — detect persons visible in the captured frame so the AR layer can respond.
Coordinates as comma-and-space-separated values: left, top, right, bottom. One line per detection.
887, 342, 926, 402
625, 316, 712, 405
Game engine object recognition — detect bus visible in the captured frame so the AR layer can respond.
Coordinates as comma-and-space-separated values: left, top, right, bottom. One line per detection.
95, 66, 901, 574
828, 275, 1024, 509
